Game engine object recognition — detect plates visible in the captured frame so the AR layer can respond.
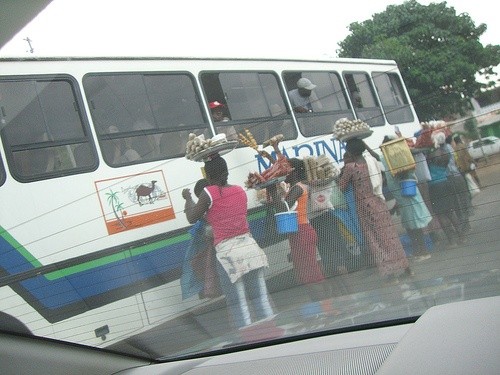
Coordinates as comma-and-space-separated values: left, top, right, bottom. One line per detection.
340, 126, 373, 142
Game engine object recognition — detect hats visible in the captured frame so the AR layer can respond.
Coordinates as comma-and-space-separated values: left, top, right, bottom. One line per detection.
209, 101, 224, 108
297, 77, 317, 90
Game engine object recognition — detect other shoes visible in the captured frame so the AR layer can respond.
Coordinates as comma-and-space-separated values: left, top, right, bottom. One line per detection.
409, 238, 475, 260
410, 272, 415, 277
383, 279, 399, 286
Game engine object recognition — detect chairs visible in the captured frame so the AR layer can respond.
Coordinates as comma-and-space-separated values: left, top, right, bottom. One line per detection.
35, 125, 121, 175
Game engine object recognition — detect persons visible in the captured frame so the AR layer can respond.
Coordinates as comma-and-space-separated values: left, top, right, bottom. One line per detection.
414, 131, 482, 248
271, 158, 324, 285
302, 162, 356, 278
182, 157, 274, 328
205, 100, 238, 146
338, 139, 414, 286
264, 104, 297, 142
340, 151, 354, 177
24, 126, 144, 173
193, 178, 214, 242
287, 78, 322, 114
379, 135, 433, 264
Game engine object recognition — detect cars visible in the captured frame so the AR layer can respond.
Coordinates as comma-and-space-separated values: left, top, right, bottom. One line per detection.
467, 136, 500, 161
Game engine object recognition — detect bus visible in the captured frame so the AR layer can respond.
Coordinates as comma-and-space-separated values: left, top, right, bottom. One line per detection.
0, 55, 436, 350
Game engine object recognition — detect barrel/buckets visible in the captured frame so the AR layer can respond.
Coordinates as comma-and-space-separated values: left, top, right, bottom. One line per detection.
274, 195, 299, 234
400, 172, 418, 197
